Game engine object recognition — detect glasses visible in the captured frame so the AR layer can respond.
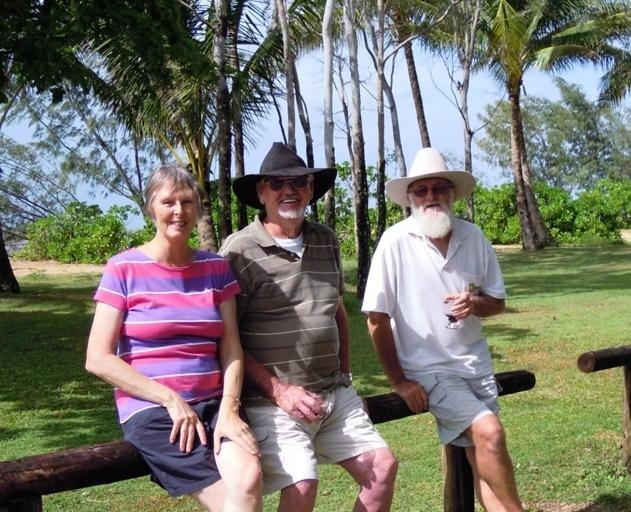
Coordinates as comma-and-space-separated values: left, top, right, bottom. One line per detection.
265, 177, 308, 191
404, 183, 452, 197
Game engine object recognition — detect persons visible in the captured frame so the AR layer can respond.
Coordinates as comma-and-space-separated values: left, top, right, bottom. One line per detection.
83, 163, 264, 511
215, 143, 399, 510
361, 145, 525, 512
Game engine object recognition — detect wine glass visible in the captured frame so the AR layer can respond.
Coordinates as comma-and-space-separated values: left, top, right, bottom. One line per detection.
441, 297, 465, 330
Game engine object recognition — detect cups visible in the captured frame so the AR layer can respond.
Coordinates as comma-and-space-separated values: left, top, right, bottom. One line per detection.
304, 388, 330, 421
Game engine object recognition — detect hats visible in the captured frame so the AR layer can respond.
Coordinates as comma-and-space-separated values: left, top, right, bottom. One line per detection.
384, 148, 476, 207
232, 142, 337, 210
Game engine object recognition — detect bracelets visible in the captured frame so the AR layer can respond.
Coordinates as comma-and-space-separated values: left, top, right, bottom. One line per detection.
224, 393, 244, 408
